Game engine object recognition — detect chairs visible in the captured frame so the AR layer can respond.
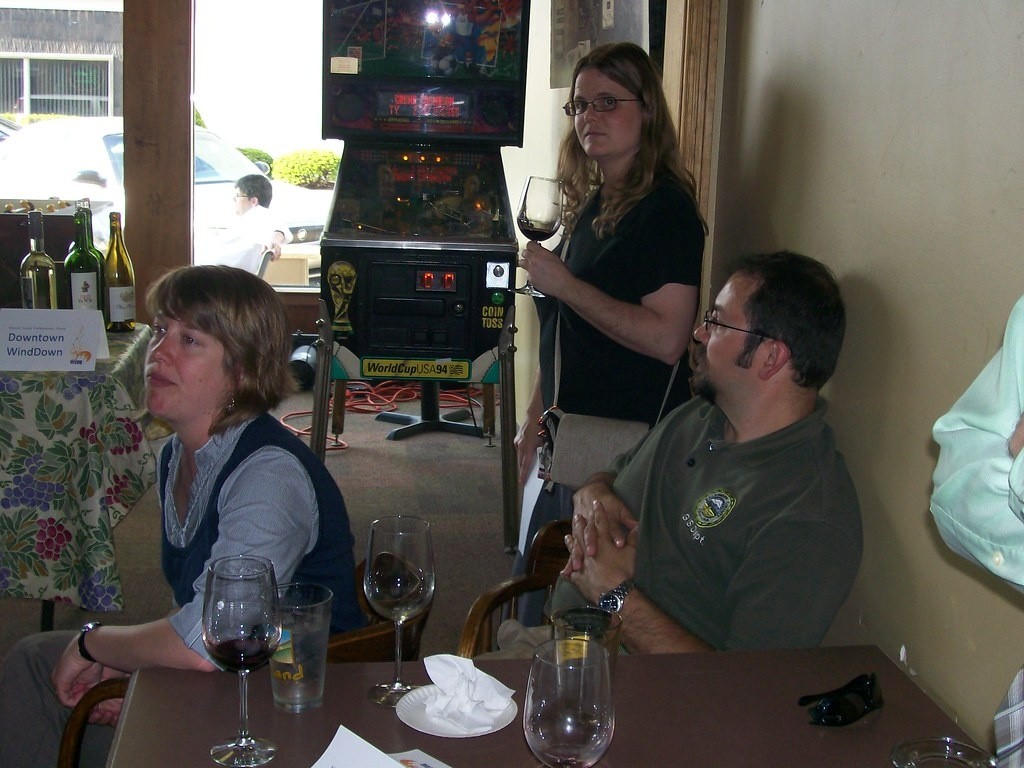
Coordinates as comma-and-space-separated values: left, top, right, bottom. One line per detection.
57, 557, 430, 768
459, 517, 577, 660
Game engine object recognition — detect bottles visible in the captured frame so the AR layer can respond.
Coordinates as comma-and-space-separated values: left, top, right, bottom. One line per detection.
105, 212, 135, 332
20, 211, 58, 310
63, 197, 104, 314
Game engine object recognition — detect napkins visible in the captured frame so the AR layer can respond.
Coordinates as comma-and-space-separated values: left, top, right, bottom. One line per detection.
423, 654, 516, 734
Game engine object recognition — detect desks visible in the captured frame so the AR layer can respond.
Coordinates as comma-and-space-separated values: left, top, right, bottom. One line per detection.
109, 646, 995, 768
0, 323, 176, 634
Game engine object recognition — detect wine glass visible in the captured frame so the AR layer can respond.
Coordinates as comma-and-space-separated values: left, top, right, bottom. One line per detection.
522, 639, 615, 768
202, 555, 280, 767
364, 515, 436, 708
506, 176, 565, 298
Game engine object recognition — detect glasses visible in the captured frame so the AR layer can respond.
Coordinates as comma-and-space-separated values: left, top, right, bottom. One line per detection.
563, 96, 643, 116
705, 306, 779, 341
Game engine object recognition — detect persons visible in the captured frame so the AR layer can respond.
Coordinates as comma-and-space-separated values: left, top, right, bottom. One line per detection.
206, 178, 290, 276
493, 42, 707, 658
929, 291, 1024, 768
53, 266, 366, 717
536, 252, 866, 660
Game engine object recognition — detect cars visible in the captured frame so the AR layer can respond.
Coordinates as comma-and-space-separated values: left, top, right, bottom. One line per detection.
0, 119, 333, 287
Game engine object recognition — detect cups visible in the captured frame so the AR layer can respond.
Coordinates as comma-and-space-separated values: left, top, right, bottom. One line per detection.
260, 582, 333, 712
550, 608, 622, 730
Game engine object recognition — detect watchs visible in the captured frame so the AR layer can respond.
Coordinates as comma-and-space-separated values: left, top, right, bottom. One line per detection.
597, 578, 637, 612
78, 622, 102, 663
